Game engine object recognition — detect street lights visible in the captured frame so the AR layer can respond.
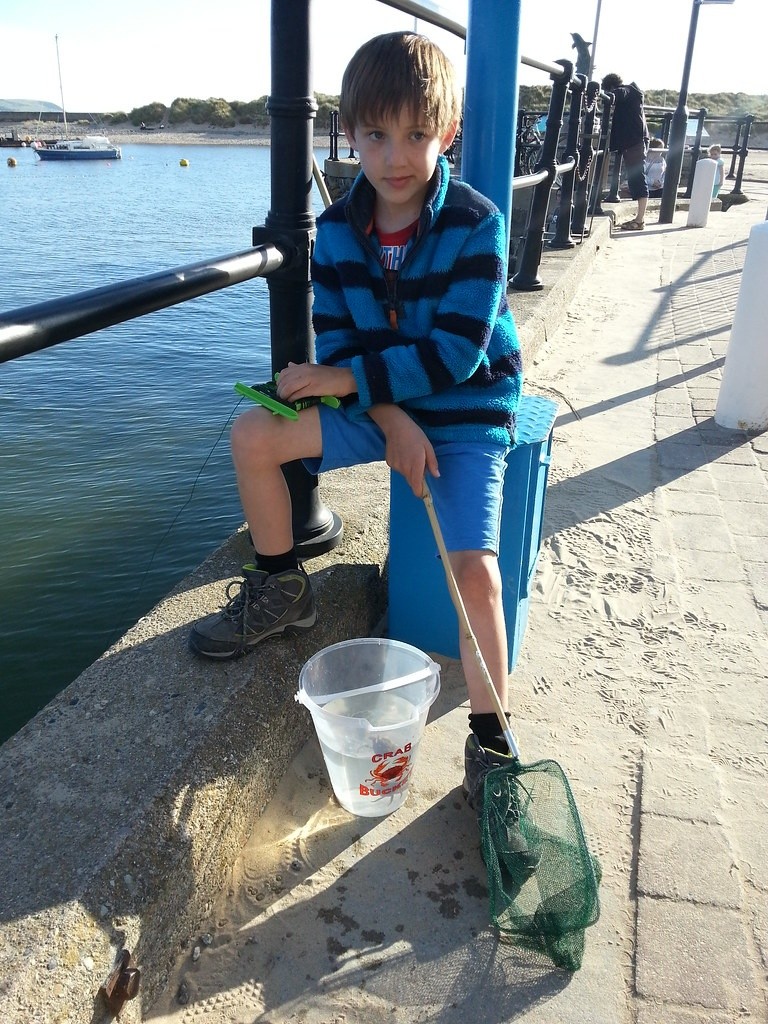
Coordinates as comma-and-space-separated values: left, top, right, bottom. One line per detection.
658, 0, 735, 224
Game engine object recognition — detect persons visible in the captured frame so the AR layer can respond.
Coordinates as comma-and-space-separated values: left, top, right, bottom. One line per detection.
709, 144, 725, 198
601, 73, 650, 231
140, 121, 146, 130
188, 31, 544, 877
644, 139, 667, 198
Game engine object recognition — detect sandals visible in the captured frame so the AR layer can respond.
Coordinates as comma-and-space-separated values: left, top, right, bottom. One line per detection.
621, 220, 644, 230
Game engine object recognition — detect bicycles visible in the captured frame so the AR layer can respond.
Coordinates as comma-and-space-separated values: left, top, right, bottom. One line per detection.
512, 105, 548, 179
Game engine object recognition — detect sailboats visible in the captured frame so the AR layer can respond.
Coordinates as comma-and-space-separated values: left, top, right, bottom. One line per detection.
28, 32, 121, 159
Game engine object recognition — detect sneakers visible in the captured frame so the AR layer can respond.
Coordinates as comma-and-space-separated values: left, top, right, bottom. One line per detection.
462, 735, 543, 876
190, 563, 317, 661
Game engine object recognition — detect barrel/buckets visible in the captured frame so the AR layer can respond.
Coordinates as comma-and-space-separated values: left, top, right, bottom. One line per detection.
293, 637, 442, 819
